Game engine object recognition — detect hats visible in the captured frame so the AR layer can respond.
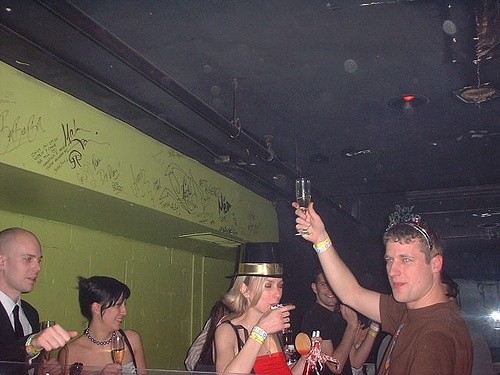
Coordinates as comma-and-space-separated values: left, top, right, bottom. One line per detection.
224, 242, 295, 278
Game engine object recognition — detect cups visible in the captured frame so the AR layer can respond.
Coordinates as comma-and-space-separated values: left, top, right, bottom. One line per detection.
271, 302, 292, 333
111, 335, 125, 364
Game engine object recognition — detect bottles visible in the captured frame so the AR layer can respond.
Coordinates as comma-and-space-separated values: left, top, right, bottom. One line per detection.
281, 329, 296, 367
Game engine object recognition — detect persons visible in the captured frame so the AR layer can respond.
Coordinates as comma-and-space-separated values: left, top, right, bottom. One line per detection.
297, 264, 385, 375
184, 242, 322, 375
57, 276, 149, 375
375, 277, 493, 375
0, 226, 79, 375
292, 201, 474, 375
349, 315, 380, 375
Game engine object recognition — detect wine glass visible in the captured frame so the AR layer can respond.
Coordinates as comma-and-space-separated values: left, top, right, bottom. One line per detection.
294, 177, 311, 235
41, 320, 55, 375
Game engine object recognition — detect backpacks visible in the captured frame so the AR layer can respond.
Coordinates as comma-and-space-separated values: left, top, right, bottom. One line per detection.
185, 300, 250, 375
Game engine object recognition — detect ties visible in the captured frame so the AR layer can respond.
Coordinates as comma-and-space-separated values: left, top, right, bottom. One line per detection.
13, 305, 24, 336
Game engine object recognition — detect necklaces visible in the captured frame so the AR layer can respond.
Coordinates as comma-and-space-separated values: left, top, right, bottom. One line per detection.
85, 327, 116, 345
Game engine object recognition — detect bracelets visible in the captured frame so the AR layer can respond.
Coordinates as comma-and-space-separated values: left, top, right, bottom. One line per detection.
369, 324, 379, 337
249, 325, 268, 344
25, 333, 43, 357
313, 236, 332, 254
